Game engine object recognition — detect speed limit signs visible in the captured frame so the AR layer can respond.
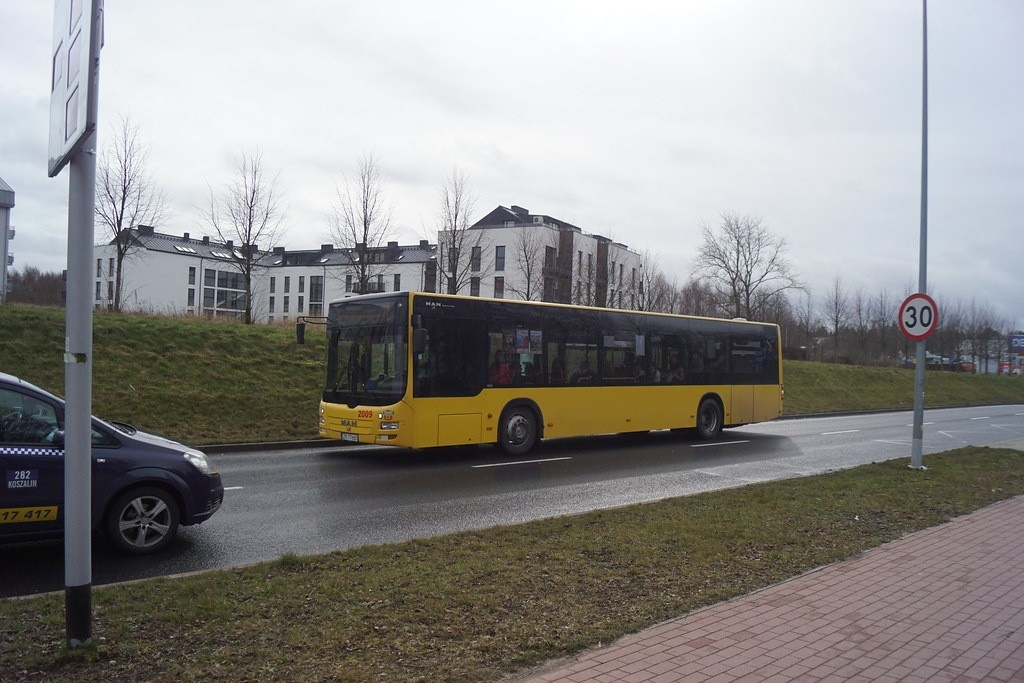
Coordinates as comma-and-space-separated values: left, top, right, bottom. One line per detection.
897, 293, 939, 341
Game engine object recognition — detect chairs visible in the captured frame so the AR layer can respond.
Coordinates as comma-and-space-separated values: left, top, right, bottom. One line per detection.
504, 344, 767, 384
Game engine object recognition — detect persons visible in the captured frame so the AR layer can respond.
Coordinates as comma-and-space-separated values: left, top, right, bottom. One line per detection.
487, 349, 513, 388
0, 408, 18, 430
392, 333, 446, 388
522, 329, 774, 384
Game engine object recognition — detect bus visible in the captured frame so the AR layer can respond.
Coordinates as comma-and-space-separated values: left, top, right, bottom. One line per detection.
297, 288, 786, 457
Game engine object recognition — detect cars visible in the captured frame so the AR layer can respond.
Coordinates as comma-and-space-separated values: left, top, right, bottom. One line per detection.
900, 350, 1023, 376
0, 374, 223, 561
